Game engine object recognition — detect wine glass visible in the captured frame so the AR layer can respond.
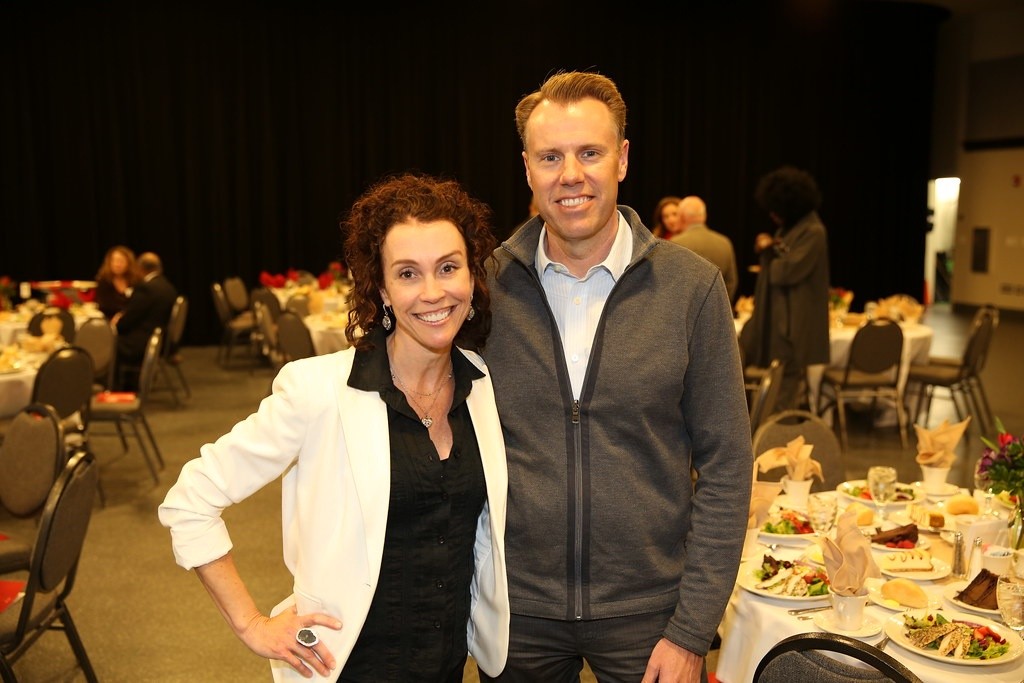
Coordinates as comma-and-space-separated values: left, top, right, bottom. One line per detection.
866, 466, 896, 522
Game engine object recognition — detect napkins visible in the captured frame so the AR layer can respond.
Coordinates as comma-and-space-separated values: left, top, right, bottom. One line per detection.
822, 505, 872, 594
757, 434, 826, 483
915, 415, 972, 467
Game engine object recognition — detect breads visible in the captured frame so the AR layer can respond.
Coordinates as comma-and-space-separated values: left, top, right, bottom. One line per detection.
952, 569, 1013, 610
881, 577, 927, 608
869, 523, 919, 547
946, 500, 979, 515
877, 549, 934, 572
845, 501, 874, 526
905, 503, 945, 527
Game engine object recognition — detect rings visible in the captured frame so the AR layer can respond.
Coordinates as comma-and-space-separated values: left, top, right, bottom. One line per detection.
296, 628, 319, 647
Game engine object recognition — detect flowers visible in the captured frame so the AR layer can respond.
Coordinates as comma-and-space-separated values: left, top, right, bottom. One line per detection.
974, 417, 1024, 537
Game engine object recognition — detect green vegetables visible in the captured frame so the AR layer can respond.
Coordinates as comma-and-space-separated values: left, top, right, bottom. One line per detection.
752, 555, 829, 596
760, 518, 809, 534
899, 612, 1009, 661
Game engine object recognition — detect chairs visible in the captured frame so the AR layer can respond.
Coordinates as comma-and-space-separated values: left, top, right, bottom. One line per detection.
0, 278, 1023, 683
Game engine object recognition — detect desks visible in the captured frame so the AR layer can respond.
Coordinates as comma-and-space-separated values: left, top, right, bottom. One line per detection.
1, 345, 48, 417
303, 310, 365, 357
730, 315, 933, 424
264, 280, 352, 310
713, 488, 1023, 683
1, 302, 106, 347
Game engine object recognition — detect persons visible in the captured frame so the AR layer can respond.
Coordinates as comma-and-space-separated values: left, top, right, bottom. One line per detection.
477, 74, 753, 683
157, 177, 510, 683
93, 246, 182, 394
748, 168, 830, 425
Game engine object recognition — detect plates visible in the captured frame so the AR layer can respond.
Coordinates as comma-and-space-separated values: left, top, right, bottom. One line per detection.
737, 477, 1024, 666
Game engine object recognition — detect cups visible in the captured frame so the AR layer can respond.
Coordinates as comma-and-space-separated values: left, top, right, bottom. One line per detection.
827, 584, 871, 630
918, 465, 951, 489
783, 474, 814, 503
995, 572, 1024, 633
981, 553, 1013, 576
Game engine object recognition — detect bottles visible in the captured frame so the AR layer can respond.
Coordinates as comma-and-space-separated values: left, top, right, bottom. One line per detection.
951, 531, 966, 578
967, 537, 983, 581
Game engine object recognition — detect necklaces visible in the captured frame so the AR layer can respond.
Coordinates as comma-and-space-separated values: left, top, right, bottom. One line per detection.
389, 356, 453, 427
